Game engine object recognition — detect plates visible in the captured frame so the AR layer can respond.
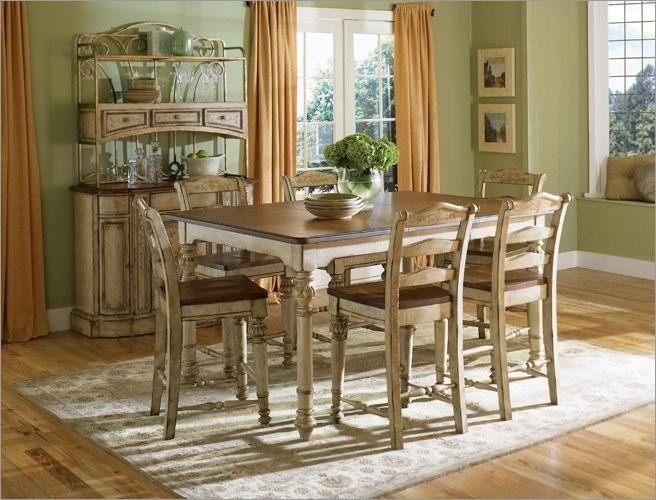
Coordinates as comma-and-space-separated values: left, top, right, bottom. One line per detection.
302, 190, 368, 222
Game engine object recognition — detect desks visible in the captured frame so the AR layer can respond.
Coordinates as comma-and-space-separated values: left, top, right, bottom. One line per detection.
142, 177, 576, 428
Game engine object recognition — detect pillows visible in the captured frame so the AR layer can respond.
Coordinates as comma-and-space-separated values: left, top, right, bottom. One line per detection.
631, 161, 655, 202
603, 153, 653, 201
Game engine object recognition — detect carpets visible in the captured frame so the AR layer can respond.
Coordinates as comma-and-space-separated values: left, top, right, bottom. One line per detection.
8, 302, 655, 499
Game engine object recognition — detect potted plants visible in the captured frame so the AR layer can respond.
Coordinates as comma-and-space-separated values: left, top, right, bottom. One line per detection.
319, 130, 401, 202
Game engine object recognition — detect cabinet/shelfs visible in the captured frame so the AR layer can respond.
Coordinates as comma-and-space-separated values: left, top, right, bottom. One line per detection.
61, 184, 272, 340
64, 13, 253, 182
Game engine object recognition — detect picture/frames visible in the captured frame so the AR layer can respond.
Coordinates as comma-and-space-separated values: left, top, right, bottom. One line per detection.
474, 43, 518, 101
475, 101, 521, 155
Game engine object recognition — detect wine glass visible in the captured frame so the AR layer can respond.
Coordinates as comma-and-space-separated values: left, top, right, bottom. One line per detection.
168, 62, 223, 103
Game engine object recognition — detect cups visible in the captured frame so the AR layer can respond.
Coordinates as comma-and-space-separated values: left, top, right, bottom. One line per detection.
126, 159, 138, 187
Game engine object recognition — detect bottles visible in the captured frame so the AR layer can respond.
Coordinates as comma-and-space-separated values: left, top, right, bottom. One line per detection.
147, 140, 162, 182
134, 147, 149, 182
145, 30, 159, 56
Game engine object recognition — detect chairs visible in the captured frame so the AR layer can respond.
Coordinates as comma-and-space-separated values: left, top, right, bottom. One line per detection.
123, 192, 279, 449
433, 187, 579, 428
277, 158, 395, 377
456, 162, 556, 367
164, 161, 269, 391
321, 195, 492, 451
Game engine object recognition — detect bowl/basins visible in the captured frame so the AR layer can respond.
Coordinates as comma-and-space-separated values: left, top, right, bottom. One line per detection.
180, 152, 225, 180
117, 75, 159, 104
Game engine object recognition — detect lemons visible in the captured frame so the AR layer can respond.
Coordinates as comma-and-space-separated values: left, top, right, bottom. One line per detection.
186, 149, 208, 159
